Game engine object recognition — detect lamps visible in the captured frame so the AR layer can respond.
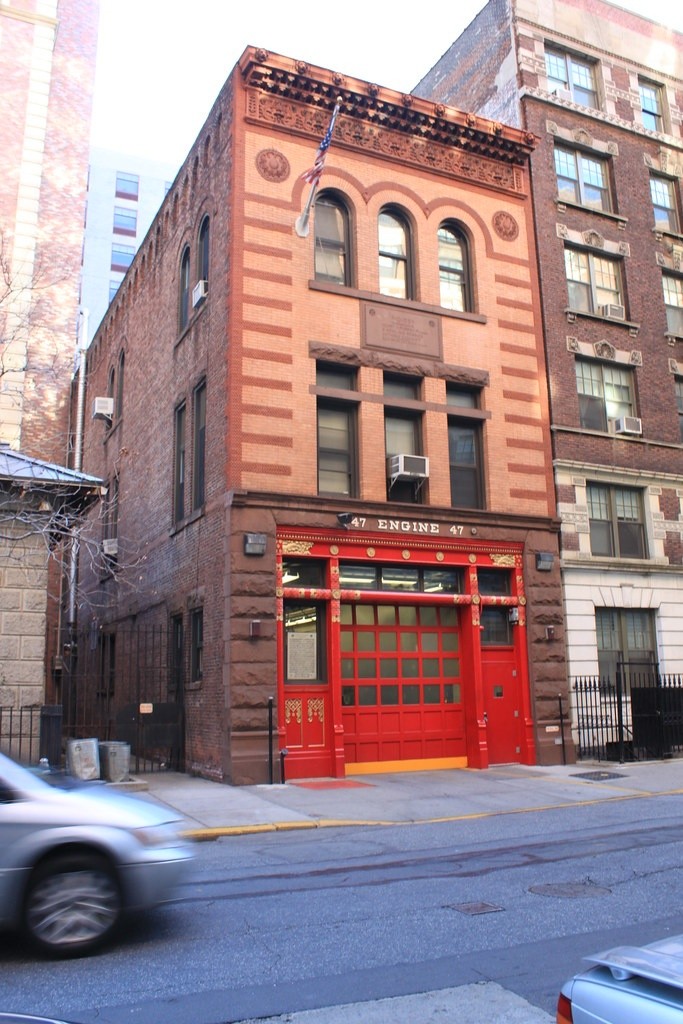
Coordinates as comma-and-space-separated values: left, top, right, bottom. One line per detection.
339, 511, 351, 526
242, 532, 268, 557
535, 551, 554, 571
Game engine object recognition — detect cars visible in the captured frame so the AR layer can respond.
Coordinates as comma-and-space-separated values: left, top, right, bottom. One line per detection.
557, 931, 683, 1024
1, 753, 192, 964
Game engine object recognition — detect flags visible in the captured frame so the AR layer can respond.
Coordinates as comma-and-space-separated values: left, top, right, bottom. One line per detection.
300, 105, 340, 187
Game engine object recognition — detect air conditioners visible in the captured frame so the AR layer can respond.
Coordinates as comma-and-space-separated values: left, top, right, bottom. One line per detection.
613, 416, 644, 435
553, 89, 574, 102
386, 454, 432, 481
98, 537, 118, 556
91, 397, 114, 419
192, 280, 208, 309
604, 303, 627, 319
